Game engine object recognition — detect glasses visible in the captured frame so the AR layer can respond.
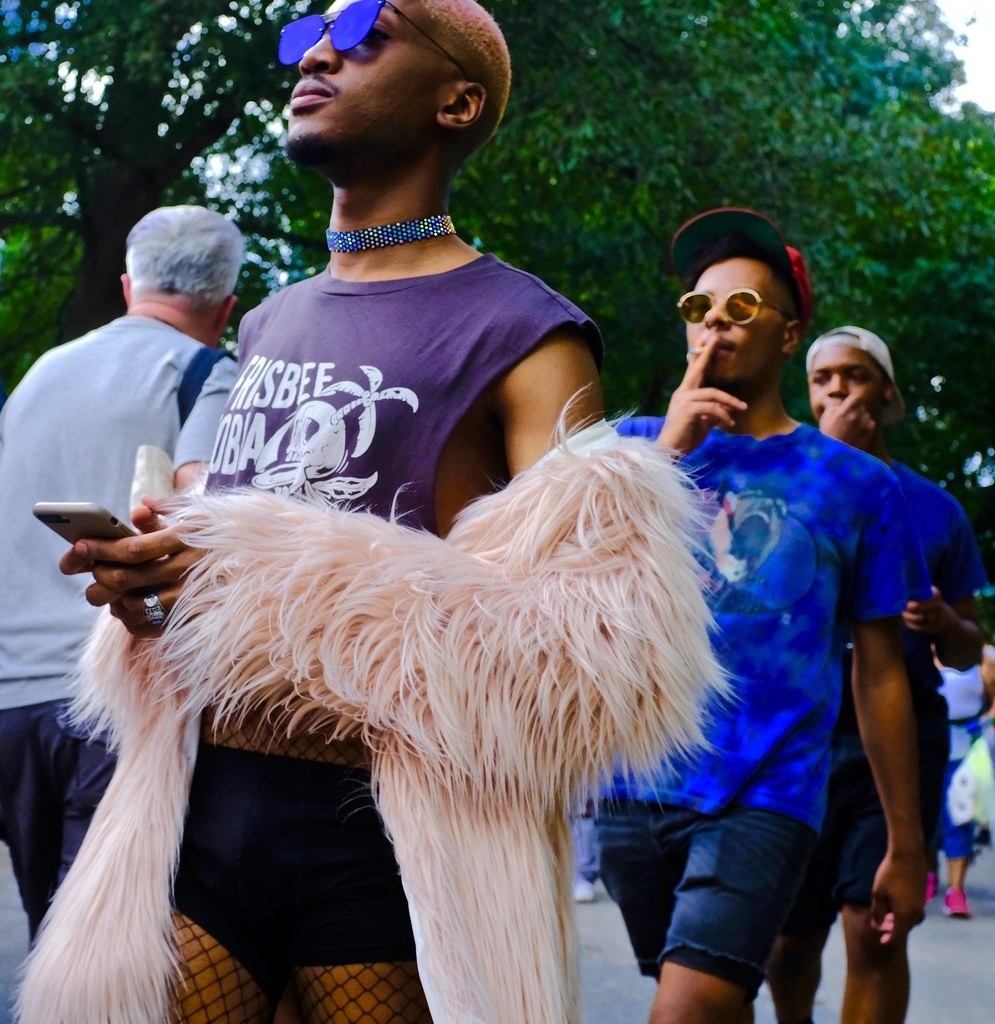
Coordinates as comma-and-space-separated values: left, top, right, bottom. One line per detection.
277, 0, 471, 83
677, 289, 795, 326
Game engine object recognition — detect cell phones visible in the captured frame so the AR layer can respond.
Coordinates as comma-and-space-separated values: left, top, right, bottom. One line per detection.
32, 502, 156, 566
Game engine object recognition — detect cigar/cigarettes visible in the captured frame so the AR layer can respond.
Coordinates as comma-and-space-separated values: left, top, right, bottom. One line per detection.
687, 347, 704, 353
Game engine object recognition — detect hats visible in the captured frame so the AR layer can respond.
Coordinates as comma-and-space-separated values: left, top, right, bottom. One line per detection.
805, 326, 904, 426
671, 208, 812, 328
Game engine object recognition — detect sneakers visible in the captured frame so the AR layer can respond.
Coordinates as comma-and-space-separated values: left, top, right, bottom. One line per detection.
924, 872, 938, 904
943, 887, 968, 917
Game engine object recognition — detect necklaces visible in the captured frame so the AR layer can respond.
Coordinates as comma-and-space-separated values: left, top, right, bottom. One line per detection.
325, 214, 458, 251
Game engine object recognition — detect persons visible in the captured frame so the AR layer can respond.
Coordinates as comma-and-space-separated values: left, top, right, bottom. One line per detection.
572, 207, 995, 1024
14, 0, 706, 1024
0, 204, 239, 953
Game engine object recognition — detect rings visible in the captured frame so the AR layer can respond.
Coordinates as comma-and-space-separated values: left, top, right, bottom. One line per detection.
144, 595, 167, 624
